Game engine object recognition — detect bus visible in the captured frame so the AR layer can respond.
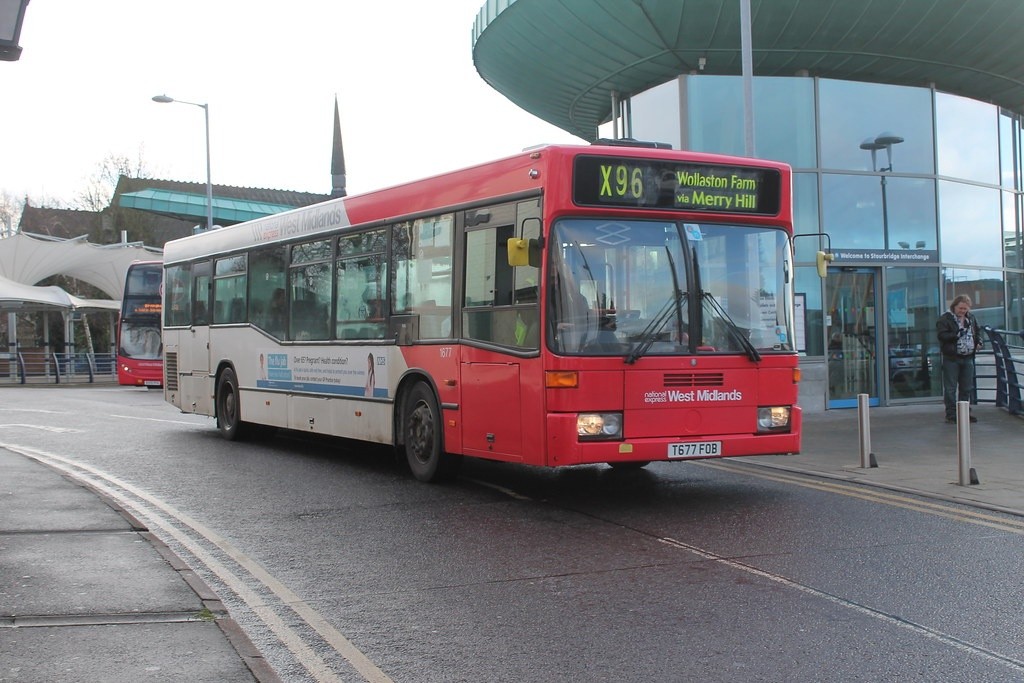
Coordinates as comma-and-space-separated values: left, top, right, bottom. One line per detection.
159, 146, 803, 484
116, 261, 163, 390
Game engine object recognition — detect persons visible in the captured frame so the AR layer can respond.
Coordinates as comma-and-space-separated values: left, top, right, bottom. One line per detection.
364, 352, 375, 398
256, 353, 266, 379
511, 255, 621, 355
935, 294, 984, 425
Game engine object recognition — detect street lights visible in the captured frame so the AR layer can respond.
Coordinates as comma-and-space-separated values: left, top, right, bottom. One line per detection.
152, 94, 212, 232
859, 131, 905, 249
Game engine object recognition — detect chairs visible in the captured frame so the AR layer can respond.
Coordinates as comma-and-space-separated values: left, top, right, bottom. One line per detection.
183, 300, 396, 340
557, 291, 623, 354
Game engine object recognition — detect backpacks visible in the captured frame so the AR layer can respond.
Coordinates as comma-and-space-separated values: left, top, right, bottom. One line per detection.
942, 311, 975, 356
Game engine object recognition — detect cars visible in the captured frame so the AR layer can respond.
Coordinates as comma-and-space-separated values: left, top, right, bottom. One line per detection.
889, 348, 932, 382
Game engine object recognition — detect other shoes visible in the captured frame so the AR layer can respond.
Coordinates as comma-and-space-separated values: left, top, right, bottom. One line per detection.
969, 416, 977, 423
946, 416, 957, 424
